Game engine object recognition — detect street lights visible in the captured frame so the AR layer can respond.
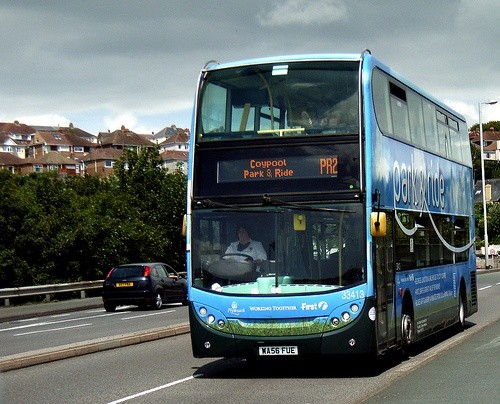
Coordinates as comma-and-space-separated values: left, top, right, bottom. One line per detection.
479, 99, 499, 271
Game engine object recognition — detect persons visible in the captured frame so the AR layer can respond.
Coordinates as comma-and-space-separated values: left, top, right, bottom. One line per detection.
221, 224, 268, 274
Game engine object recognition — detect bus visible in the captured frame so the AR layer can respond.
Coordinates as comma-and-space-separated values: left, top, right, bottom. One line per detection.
181, 48, 480, 364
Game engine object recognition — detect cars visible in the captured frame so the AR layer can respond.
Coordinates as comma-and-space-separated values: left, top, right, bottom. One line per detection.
101, 261, 189, 313
